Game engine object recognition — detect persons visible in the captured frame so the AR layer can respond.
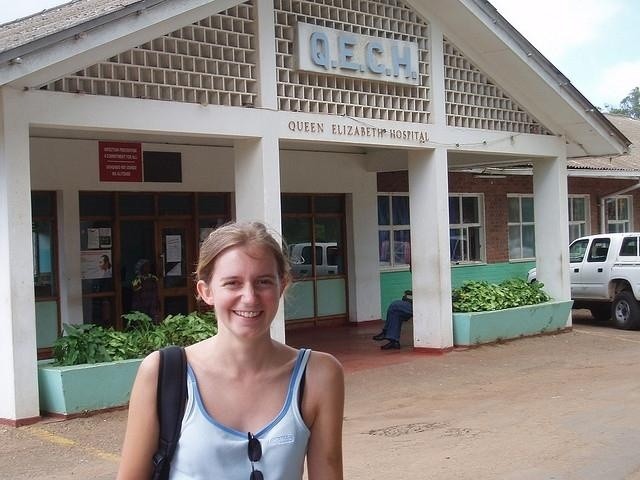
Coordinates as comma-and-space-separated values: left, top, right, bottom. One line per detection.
122, 258, 160, 315
115, 220, 346, 480
99, 254, 111, 272
372, 289, 412, 350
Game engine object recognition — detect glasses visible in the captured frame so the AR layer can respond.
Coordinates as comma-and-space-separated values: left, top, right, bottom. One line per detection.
248, 432, 264, 480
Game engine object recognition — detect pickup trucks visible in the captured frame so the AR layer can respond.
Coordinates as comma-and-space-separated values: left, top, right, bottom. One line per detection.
526, 234, 640, 330
282, 242, 339, 276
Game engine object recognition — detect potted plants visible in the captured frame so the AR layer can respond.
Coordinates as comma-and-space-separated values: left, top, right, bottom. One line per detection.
453, 276, 575, 347
38, 311, 218, 420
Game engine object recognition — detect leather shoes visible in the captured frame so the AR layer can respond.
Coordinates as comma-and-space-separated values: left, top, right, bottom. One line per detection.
373, 329, 388, 339
381, 340, 400, 350
